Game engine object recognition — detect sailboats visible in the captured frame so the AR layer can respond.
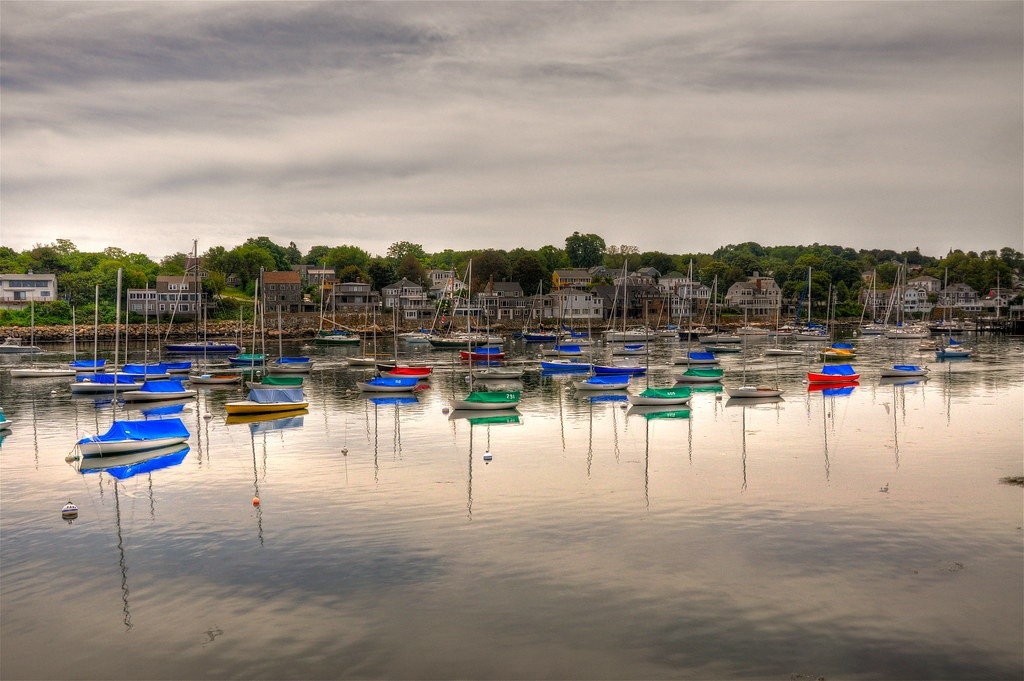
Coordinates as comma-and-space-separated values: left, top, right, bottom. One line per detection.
0, 238, 316, 415
448, 259, 520, 408
69, 443, 190, 630
878, 375, 930, 470
725, 395, 785, 492
807, 383, 860, 482
312, 257, 964, 394
626, 301, 693, 405
77, 280, 191, 459
725, 290, 786, 398
448, 410, 523, 515
626, 404, 692, 511
224, 409, 309, 548
